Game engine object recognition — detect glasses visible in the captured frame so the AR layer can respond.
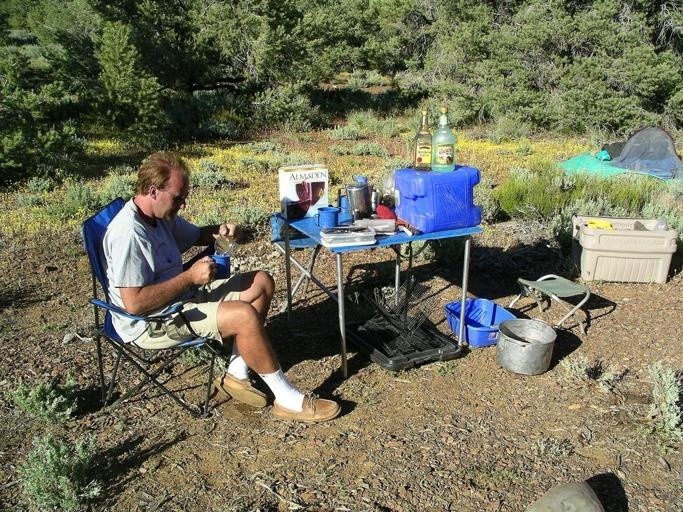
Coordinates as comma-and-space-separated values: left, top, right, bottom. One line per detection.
169, 193, 183, 202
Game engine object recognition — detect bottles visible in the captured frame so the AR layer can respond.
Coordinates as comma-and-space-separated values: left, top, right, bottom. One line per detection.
432, 106, 458, 173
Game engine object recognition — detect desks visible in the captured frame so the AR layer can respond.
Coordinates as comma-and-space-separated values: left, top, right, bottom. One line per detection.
273, 211, 485, 380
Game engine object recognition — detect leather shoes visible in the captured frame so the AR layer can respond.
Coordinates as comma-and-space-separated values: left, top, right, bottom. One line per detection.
213, 371, 268, 407
272, 393, 339, 422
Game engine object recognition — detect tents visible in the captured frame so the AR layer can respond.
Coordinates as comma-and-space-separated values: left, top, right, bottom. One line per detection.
608, 126, 681, 181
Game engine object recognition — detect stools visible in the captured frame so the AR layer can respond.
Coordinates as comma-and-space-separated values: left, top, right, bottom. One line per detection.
509, 274, 590, 334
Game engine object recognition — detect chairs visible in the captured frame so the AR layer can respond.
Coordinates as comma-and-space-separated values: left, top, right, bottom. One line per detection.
78, 196, 231, 418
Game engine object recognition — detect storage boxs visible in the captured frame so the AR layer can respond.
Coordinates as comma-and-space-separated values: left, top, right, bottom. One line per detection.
443, 297, 518, 348
571, 214, 678, 285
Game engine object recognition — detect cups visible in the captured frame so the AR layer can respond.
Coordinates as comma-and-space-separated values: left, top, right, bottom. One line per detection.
209, 255, 230, 280
338, 182, 374, 220
372, 188, 383, 214
313, 207, 340, 229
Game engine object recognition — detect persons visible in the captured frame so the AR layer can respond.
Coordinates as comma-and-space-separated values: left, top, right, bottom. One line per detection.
103, 150, 342, 423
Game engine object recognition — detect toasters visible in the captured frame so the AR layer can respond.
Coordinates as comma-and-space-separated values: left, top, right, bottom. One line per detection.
411, 110, 432, 173
